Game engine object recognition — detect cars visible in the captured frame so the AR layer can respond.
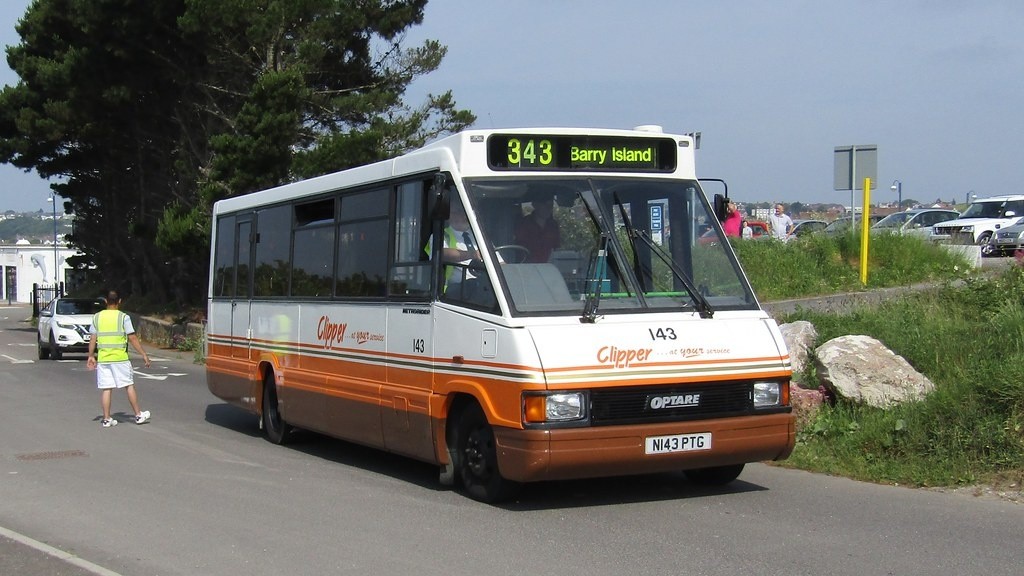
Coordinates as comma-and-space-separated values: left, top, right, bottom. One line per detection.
786, 219, 828, 245
990, 217, 1024, 256
745, 221, 768, 239
823, 213, 885, 236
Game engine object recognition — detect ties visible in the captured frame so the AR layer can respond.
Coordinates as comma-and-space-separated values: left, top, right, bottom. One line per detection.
461, 231, 475, 253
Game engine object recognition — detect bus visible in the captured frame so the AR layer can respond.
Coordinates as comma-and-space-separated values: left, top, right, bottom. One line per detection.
203, 123, 798, 510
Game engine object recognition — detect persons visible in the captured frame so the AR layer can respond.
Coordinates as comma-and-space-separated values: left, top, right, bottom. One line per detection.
87, 291, 151, 428
724, 202, 741, 237
742, 221, 752, 240
516, 192, 561, 264
424, 200, 507, 294
766, 204, 792, 238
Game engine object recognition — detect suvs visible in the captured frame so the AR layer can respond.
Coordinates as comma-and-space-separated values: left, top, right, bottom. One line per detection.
870, 208, 961, 242
37, 295, 129, 361
931, 195, 1024, 257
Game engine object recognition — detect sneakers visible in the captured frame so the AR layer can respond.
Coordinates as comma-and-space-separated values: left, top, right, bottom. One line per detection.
102, 417, 118, 427
134, 410, 150, 424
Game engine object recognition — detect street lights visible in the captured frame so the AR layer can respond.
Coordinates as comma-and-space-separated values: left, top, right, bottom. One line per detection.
890, 179, 902, 212
47, 191, 60, 296
966, 190, 978, 206
